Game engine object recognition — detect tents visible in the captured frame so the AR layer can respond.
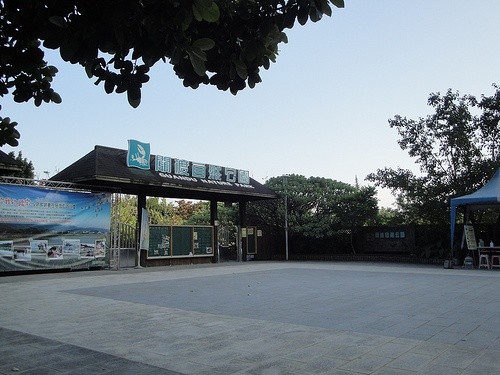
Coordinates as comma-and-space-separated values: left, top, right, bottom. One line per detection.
450, 167, 500, 269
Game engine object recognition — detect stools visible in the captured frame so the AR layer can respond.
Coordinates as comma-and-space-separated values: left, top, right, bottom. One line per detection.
479, 254, 490, 270
491, 255, 500, 270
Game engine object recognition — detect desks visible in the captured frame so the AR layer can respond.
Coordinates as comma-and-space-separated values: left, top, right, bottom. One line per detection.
476, 246, 500, 258
